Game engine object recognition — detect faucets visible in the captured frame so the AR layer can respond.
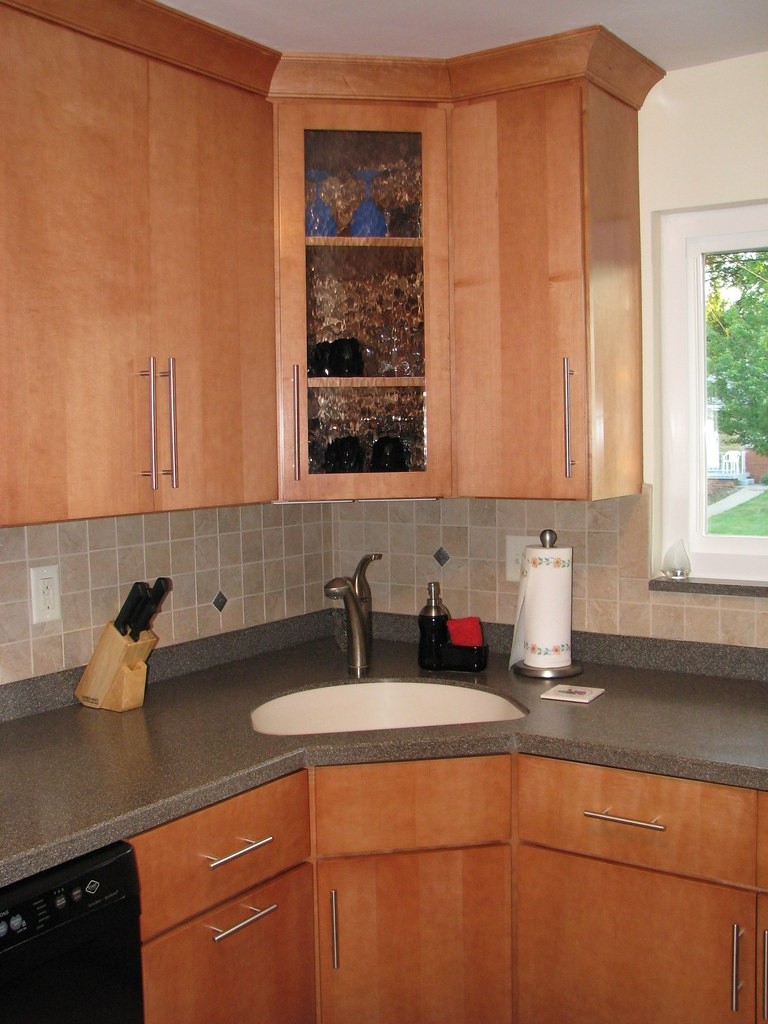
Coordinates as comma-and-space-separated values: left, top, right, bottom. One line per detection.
321, 577, 375, 679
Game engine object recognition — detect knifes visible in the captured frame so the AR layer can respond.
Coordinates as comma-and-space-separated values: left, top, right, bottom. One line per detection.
114, 577, 167, 642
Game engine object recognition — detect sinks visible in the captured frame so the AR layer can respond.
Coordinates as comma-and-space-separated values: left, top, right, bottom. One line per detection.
246, 665, 533, 737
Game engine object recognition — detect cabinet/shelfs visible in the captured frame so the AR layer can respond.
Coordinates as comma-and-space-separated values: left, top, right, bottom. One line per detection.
274, 100, 449, 500
513, 753, 768, 1024
125, 767, 316, 1021
448, 78, 643, 501
0, 0, 282, 526
310, 754, 516, 1022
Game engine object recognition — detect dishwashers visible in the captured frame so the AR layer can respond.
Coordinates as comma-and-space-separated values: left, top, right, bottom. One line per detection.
0, 840, 144, 1024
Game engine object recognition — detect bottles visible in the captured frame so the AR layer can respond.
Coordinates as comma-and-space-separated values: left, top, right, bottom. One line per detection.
417, 583, 450, 670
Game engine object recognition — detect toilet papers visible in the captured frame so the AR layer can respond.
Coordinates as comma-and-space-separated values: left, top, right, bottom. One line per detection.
508, 542, 575, 672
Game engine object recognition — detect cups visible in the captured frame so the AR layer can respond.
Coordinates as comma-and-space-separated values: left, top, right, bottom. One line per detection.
452, 643, 489, 672
